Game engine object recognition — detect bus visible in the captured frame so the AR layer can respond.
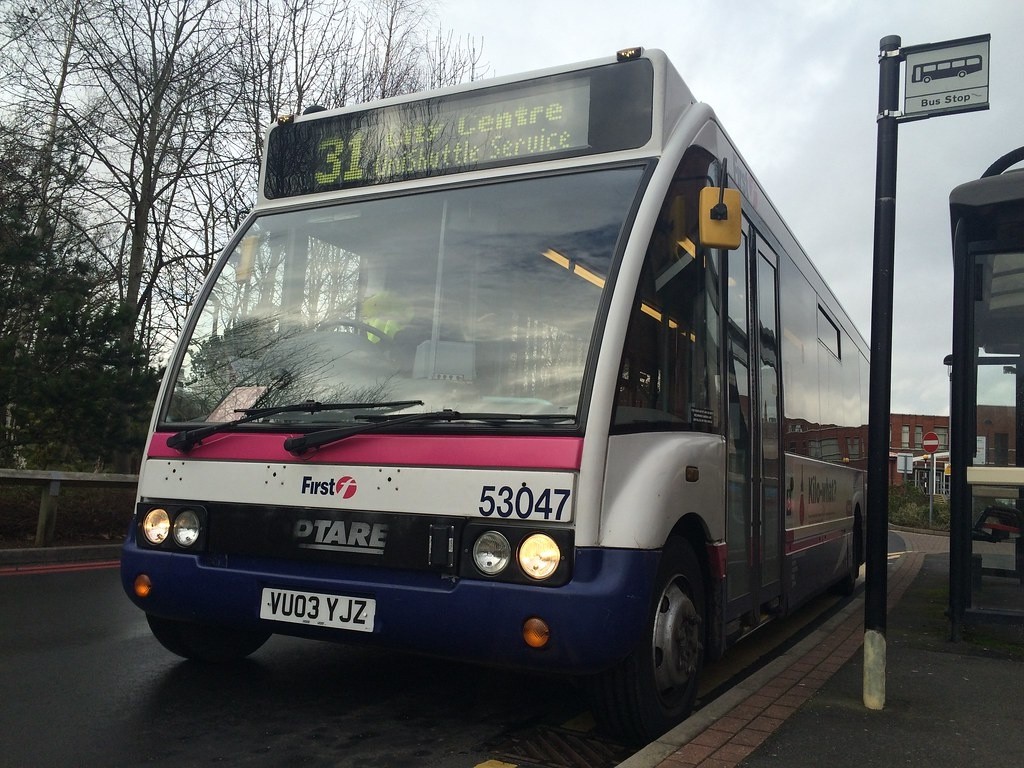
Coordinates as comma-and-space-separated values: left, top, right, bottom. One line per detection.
119, 47, 874, 750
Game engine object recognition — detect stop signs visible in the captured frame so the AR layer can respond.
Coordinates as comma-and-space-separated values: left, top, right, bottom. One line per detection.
921, 432, 939, 454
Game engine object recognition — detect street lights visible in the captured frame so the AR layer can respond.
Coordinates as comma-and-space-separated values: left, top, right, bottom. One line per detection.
984, 418, 992, 464
